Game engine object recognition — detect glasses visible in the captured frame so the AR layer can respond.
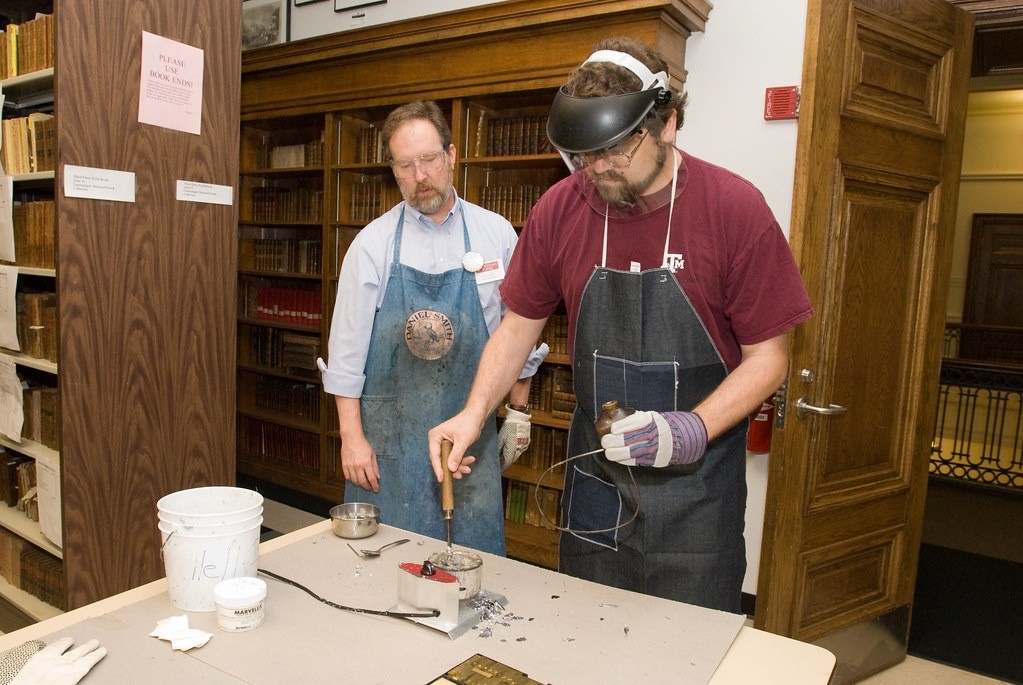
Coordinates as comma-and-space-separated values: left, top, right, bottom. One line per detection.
389, 145, 449, 179
568, 130, 650, 170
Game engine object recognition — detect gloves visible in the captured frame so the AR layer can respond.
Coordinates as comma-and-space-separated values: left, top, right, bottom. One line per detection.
601, 410, 708, 467
498, 403, 532, 472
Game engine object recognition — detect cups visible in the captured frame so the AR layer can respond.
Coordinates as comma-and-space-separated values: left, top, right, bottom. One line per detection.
427, 550, 483, 600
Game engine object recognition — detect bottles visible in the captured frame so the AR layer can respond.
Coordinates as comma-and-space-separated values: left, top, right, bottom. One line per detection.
594, 400, 635, 450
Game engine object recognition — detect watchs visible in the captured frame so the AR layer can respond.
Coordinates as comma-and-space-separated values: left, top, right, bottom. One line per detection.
508, 404, 531, 415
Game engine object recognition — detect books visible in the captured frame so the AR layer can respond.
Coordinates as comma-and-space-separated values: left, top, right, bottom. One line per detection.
349, 126, 405, 221
236, 128, 326, 471
475, 111, 578, 532
0, 15, 68, 614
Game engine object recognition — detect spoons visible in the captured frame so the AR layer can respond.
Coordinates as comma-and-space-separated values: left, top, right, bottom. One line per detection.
360, 539, 410, 557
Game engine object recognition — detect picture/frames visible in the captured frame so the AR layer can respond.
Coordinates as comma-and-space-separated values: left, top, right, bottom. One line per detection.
243, 0, 291, 50
334, 0, 387, 13
293, 0, 329, 7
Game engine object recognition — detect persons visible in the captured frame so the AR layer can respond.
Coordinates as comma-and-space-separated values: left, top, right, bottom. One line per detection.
428, 38, 816, 616
317, 103, 550, 558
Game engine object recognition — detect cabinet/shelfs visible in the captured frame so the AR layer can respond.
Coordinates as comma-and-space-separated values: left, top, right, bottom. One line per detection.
235, 0, 715, 572
0, 0, 244, 623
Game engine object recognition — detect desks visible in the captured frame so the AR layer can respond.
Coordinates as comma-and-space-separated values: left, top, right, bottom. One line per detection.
0, 519, 838, 685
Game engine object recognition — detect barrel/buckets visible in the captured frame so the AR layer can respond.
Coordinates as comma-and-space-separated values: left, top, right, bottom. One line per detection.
158, 485, 264, 613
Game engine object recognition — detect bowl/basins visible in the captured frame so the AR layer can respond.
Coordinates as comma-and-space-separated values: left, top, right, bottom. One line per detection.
329, 502, 381, 539
213, 576, 267, 632
